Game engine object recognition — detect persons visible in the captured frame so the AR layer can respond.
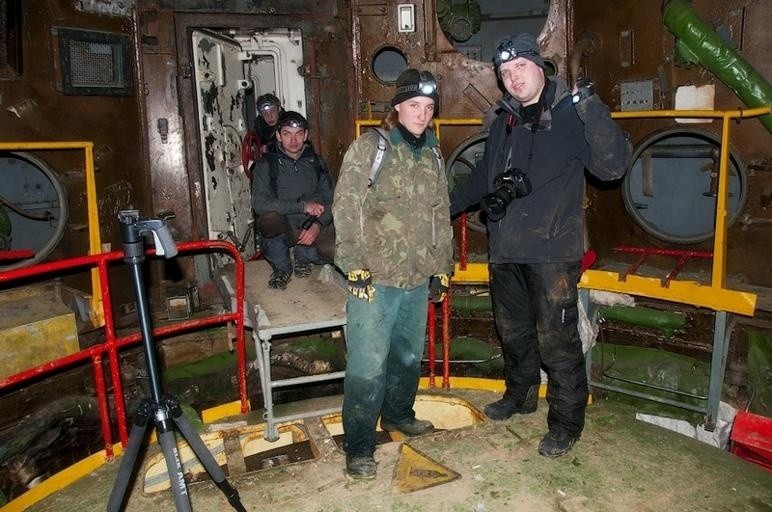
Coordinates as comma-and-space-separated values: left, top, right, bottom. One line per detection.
331, 69, 454, 478
240, 93, 335, 289
448, 36, 634, 458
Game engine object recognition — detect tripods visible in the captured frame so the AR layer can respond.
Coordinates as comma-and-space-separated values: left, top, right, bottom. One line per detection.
107, 210, 248, 512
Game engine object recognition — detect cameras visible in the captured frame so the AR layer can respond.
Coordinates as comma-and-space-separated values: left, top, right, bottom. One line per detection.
479, 167, 532, 223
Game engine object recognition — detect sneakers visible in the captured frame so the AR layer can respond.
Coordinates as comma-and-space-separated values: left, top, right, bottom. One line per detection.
293, 248, 313, 278
483, 396, 540, 420
379, 415, 435, 437
538, 422, 582, 458
346, 447, 378, 480
267, 268, 292, 290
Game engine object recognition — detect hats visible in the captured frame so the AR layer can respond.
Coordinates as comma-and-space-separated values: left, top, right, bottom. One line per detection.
492, 32, 547, 79
256, 93, 282, 111
279, 111, 308, 128
392, 69, 439, 115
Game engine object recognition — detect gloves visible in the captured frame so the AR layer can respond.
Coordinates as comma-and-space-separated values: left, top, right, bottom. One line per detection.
344, 267, 376, 303
571, 75, 597, 105
428, 272, 450, 304
